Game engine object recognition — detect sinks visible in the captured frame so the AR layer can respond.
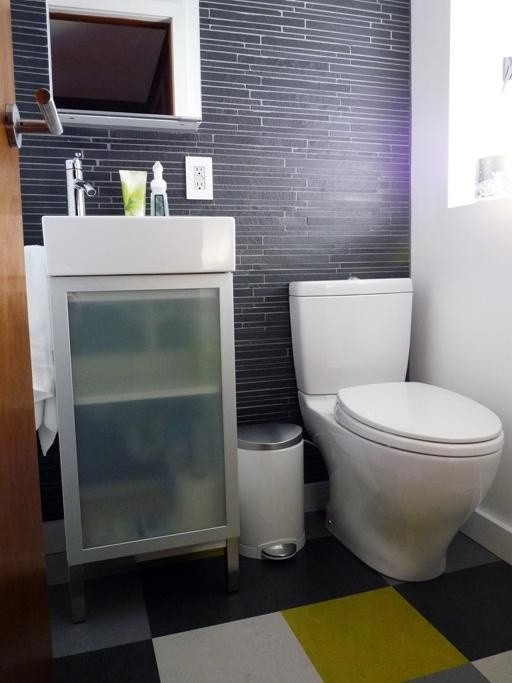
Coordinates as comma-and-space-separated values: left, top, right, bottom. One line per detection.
42, 215, 236, 277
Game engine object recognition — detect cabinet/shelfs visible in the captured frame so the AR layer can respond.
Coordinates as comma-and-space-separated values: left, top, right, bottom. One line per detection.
45, 271, 243, 626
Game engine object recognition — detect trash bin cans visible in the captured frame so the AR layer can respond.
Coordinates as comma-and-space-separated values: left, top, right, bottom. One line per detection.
238, 422, 306, 559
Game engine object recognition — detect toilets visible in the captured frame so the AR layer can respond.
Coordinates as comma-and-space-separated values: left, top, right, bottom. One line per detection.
289, 277, 505, 582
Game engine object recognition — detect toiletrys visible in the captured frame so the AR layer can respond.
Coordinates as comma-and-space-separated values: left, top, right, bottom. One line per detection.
118, 170, 147, 216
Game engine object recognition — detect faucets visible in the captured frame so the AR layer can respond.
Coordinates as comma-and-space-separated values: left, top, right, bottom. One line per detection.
66, 152, 96, 216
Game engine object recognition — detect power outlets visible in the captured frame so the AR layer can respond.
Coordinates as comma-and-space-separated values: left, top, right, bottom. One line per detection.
185, 156, 215, 198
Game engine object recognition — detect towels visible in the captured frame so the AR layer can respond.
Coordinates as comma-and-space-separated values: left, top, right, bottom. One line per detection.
23, 245, 58, 456
